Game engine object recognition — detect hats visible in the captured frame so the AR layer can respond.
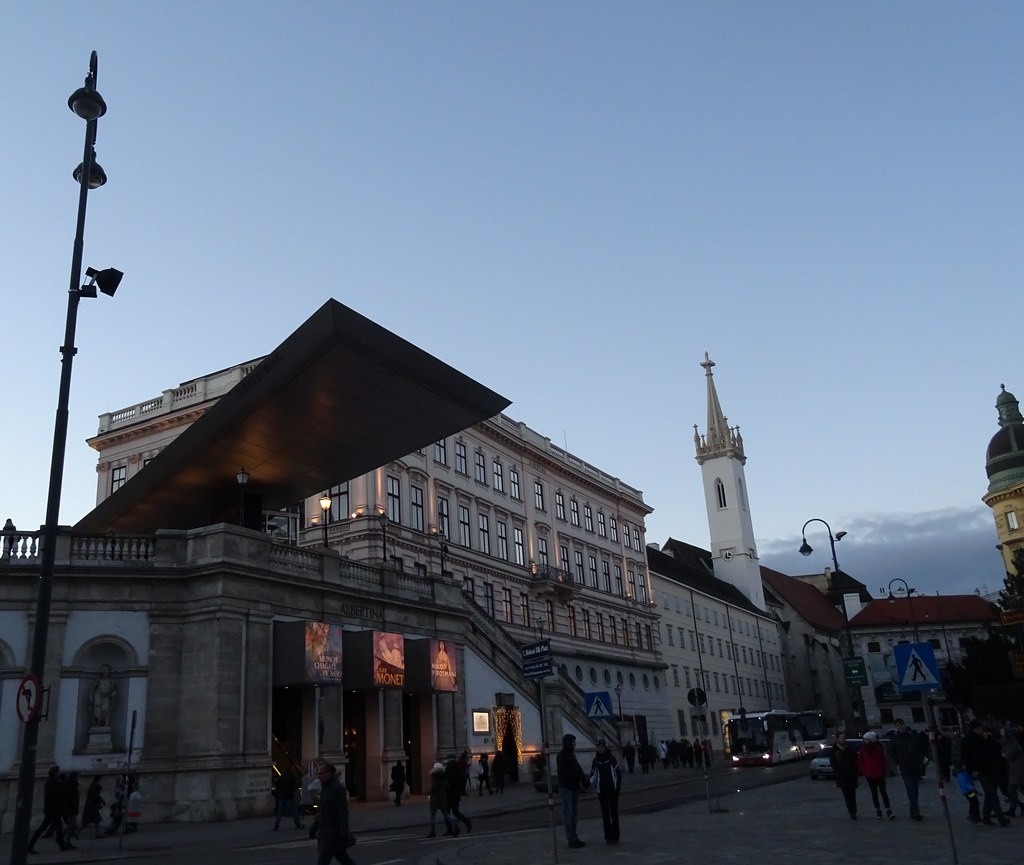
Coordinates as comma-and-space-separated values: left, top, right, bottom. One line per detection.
595, 739, 606, 746
428, 763, 446, 775
863, 731, 877, 742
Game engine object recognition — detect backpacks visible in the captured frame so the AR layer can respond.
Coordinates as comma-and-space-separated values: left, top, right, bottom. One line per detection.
476, 763, 484, 775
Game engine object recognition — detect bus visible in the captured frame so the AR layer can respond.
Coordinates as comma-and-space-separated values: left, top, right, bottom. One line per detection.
722, 709, 830, 768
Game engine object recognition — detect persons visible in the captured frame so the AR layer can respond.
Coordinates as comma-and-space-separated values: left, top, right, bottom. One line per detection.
74, 775, 106, 838
587, 740, 622, 844
637, 738, 712, 774
556, 734, 589, 847
27, 765, 79, 855
272, 753, 305, 831
309, 763, 357, 865
426, 762, 460, 836
90, 663, 116, 726
623, 742, 635, 773
906, 724, 952, 783
893, 719, 924, 820
105, 775, 137, 835
949, 718, 1024, 827
458, 751, 505, 795
858, 732, 895, 820
830, 732, 858, 820
391, 761, 405, 805
440, 753, 471, 836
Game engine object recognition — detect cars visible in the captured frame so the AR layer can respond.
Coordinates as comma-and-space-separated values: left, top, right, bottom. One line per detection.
810, 727, 893, 781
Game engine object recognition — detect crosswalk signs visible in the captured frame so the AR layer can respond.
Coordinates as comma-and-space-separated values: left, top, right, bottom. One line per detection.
585, 691, 613, 720
892, 642, 944, 694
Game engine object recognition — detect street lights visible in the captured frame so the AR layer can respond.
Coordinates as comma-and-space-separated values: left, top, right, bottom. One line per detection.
437, 529, 447, 577
798, 518, 869, 733
11, 50, 126, 865
378, 512, 390, 563
614, 683, 622, 722
536, 617, 547, 641
318, 493, 332, 548
235, 466, 251, 528
886, 578, 939, 746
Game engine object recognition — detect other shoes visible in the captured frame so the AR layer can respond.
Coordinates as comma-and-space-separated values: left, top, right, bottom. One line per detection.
1004, 809, 1017, 817
850, 814, 857, 820
25, 826, 148, 857
1002, 819, 1011, 826
425, 818, 472, 838
910, 813, 923, 821
604, 835, 619, 845
875, 808, 883, 818
983, 820, 994, 825
478, 787, 504, 796
966, 816, 978, 824
393, 798, 401, 807
293, 823, 303, 828
271, 826, 278, 829
886, 809, 894, 819
567, 839, 586, 848
